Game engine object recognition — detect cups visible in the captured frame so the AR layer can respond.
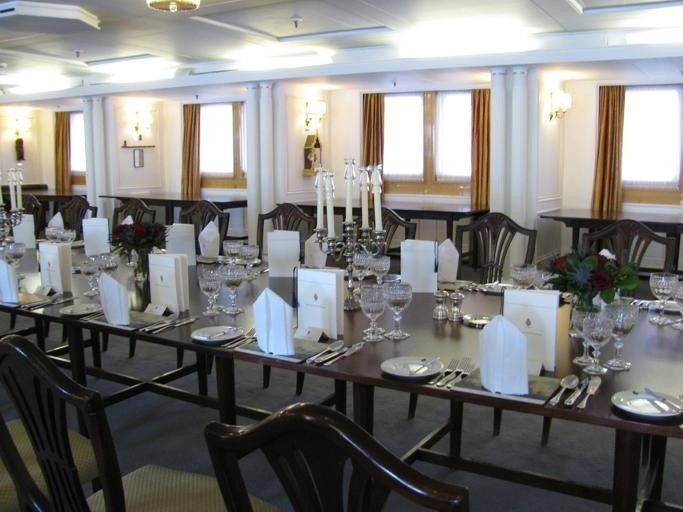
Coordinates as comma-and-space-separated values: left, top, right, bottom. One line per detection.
510, 263, 543, 289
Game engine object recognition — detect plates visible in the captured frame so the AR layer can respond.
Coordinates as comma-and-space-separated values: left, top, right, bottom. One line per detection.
438, 280, 511, 293
380, 356, 443, 379
613, 390, 683, 418
191, 326, 244, 345
463, 312, 493, 327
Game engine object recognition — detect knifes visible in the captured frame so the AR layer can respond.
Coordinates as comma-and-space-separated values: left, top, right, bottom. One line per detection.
408, 355, 439, 375
324, 341, 363, 366
136, 313, 195, 333
644, 387, 682, 414
578, 375, 601, 412
564, 376, 588, 406
314, 345, 348, 364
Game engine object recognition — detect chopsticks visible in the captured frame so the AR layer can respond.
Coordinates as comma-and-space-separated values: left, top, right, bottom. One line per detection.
548, 374, 578, 406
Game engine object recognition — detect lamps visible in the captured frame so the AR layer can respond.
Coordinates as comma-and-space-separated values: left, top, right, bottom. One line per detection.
0, 0, 102, 35
146, 0, 203, 13
550, 90, 572, 124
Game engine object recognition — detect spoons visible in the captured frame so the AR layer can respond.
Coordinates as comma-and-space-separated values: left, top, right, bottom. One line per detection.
305, 339, 343, 364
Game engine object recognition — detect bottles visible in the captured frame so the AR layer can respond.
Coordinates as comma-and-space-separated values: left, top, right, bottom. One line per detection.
431, 286, 447, 320
446, 290, 464, 323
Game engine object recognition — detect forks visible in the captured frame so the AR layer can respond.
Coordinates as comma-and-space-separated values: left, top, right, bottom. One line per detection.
427, 358, 475, 388
220, 327, 255, 348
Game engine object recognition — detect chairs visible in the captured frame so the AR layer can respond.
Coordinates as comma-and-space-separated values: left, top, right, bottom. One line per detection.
1, 192, 682, 511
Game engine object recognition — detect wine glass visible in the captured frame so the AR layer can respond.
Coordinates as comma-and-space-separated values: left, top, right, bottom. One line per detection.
570, 299, 639, 375
648, 272, 682, 330
354, 253, 412, 342
0, 221, 259, 313
